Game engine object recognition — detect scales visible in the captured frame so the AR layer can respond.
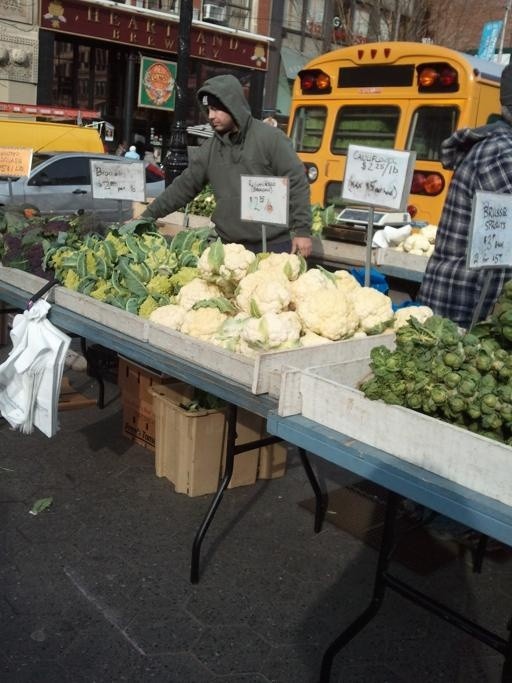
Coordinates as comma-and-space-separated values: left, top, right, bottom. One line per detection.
334, 204, 411, 227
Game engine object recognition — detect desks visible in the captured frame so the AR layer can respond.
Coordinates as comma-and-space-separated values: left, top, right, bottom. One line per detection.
305, 251, 427, 284
263, 415, 512, 683
1, 280, 330, 584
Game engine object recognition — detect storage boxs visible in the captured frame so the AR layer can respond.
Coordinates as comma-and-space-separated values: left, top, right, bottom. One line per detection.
121, 404, 155, 453
148, 381, 289, 499
117, 352, 184, 421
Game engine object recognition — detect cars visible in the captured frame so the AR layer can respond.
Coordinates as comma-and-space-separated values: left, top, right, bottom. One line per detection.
0, 149, 171, 228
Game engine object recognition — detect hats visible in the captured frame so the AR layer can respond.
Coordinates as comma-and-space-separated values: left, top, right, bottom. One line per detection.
199, 94, 229, 113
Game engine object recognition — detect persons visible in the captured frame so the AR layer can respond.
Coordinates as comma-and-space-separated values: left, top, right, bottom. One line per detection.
129, 71, 315, 264
412, 59, 512, 335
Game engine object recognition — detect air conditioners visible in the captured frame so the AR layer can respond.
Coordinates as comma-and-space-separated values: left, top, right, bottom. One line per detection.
203, 4, 225, 22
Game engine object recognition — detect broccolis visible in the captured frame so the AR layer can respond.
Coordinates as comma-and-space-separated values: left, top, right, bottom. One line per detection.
39, 220, 437, 358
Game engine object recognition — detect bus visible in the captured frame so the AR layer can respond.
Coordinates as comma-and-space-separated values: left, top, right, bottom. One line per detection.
284, 42, 507, 228
